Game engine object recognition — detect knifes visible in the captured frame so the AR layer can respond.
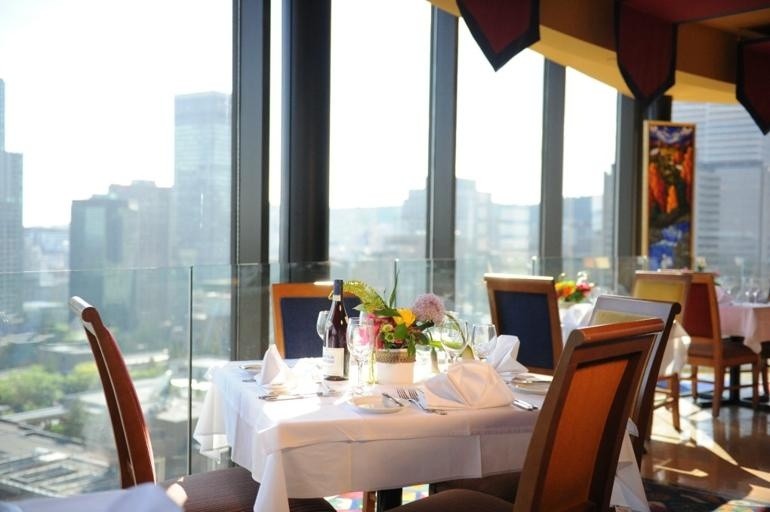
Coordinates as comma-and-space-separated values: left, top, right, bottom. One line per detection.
507, 378, 552, 387
382, 391, 402, 410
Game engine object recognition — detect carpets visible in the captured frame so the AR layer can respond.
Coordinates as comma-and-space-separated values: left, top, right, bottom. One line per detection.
473, 472, 769, 512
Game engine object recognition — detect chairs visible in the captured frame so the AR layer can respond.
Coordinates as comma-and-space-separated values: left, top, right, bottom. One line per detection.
381, 311, 664, 512
70, 294, 338, 512
629, 273, 691, 442
680, 274, 758, 417
479, 271, 562, 374
270, 281, 365, 358
586, 294, 682, 472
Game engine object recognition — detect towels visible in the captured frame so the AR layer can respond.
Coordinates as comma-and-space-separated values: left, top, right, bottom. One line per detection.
414, 356, 514, 411
252, 343, 288, 386
485, 334, 529, 376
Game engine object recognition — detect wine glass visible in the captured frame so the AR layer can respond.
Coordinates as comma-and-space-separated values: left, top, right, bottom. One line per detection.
345, 314, 374, 394
360, 313, 384, 384
437, 319, 468, 363
723, 276, 761, 306
315, 308, 330, 368
470, 324, 498, 361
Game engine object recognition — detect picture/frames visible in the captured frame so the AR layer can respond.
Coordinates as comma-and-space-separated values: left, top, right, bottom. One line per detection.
641, 119, 697, 273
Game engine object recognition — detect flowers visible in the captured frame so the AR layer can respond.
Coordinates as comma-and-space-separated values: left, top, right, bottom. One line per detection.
554, 278, 594, 304
351, 259, 466, 355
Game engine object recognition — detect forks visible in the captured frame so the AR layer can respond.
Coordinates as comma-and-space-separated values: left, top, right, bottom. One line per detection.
408, 387, 426, 412
395, 385, 427, 415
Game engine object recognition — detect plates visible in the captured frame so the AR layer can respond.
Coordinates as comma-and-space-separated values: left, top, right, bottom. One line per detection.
504, 372, 555, 398
347, 393, 410, 413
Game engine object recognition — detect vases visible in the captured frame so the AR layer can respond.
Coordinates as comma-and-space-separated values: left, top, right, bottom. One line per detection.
374, 348, 416, 388
558, 300, 576, 311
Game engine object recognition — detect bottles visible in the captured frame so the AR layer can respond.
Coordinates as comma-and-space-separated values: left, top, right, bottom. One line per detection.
323, 279, 348, 383
